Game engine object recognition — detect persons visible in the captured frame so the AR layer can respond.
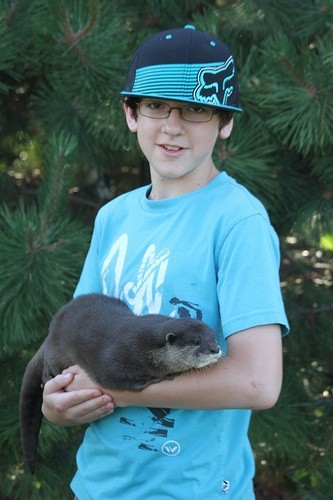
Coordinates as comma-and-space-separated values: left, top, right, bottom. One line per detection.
40, 24, 291, 500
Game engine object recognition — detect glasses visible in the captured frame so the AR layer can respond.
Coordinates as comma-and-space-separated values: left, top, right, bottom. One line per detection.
131, 101, 219, 123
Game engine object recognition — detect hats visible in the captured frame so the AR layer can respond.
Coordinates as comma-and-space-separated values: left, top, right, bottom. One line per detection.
119, 24, 243, 114
